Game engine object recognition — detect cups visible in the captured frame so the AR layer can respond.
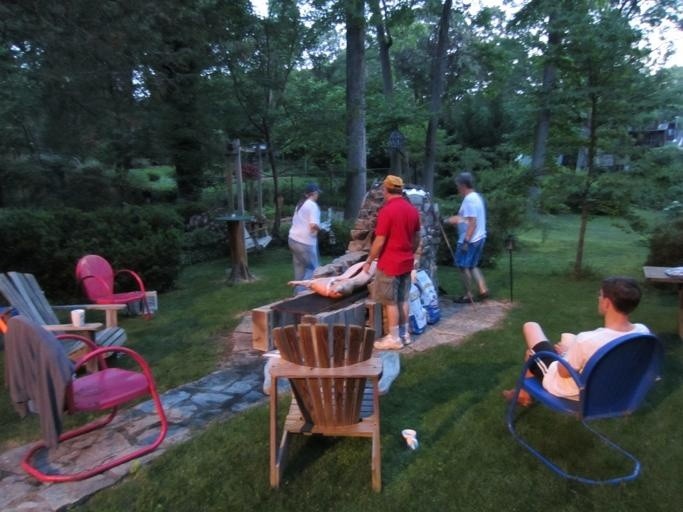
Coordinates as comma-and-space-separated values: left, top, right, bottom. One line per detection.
70, 308, 86, 325
401, 429, 418, 449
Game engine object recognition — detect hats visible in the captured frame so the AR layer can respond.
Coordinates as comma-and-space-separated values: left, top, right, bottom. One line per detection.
304, 184, 321, 194
383, 175, 403, 191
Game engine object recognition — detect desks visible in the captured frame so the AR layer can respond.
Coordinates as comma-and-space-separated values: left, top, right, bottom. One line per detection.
214, 213, 257, 287
640, 262, 682, 340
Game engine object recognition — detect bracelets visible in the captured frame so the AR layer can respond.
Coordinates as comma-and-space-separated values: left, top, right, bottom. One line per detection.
366, 256, 373, 265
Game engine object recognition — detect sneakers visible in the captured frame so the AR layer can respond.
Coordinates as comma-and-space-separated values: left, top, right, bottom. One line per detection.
372, 333, 404, 350
400, 333, 410, 345
502, 389, 532, 407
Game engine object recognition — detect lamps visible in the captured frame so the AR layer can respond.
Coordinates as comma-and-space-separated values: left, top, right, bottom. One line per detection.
503, 233, 521, 306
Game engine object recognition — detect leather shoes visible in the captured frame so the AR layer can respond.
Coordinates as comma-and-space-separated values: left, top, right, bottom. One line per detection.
473, 293, 488, 301
452, 296, 470, 303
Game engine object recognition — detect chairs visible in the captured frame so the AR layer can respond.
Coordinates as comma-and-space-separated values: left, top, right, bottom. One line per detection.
260, 322, 401, 493
3, 315, 169, 485
73, 253, 157, 325
1, 271, 131, 416
504, 326, 666, 489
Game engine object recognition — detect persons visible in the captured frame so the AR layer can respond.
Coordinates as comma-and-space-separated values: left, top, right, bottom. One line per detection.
362, 174, 420, 350
286, 184, 331, 296
439, 172, 488, 304
502, 276, 652, 409
